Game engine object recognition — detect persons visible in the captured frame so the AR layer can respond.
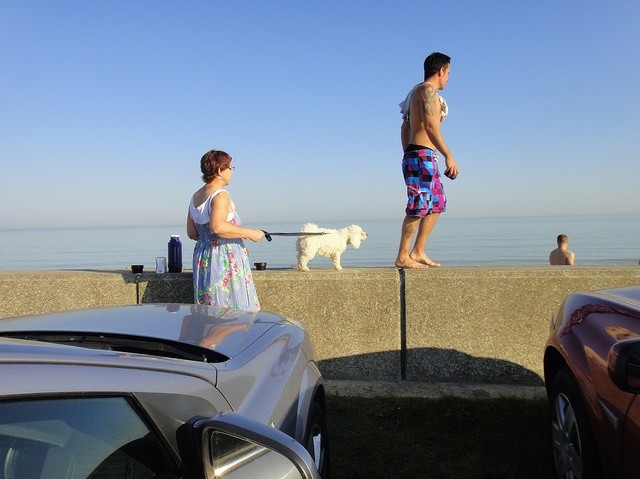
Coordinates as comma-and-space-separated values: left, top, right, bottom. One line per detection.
549, 234, 574, 265
394, 52, 459, 270
187, 150, 266, 312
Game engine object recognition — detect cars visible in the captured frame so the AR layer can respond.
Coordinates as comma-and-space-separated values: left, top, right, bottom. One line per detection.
0, 302, 329, 478
544, 287, 640, 478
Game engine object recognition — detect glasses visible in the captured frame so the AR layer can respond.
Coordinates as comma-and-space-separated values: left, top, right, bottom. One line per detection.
227, 165, 235, 174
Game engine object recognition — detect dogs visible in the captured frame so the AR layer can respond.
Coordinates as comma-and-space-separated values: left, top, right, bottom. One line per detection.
296, 222, 368, 271
